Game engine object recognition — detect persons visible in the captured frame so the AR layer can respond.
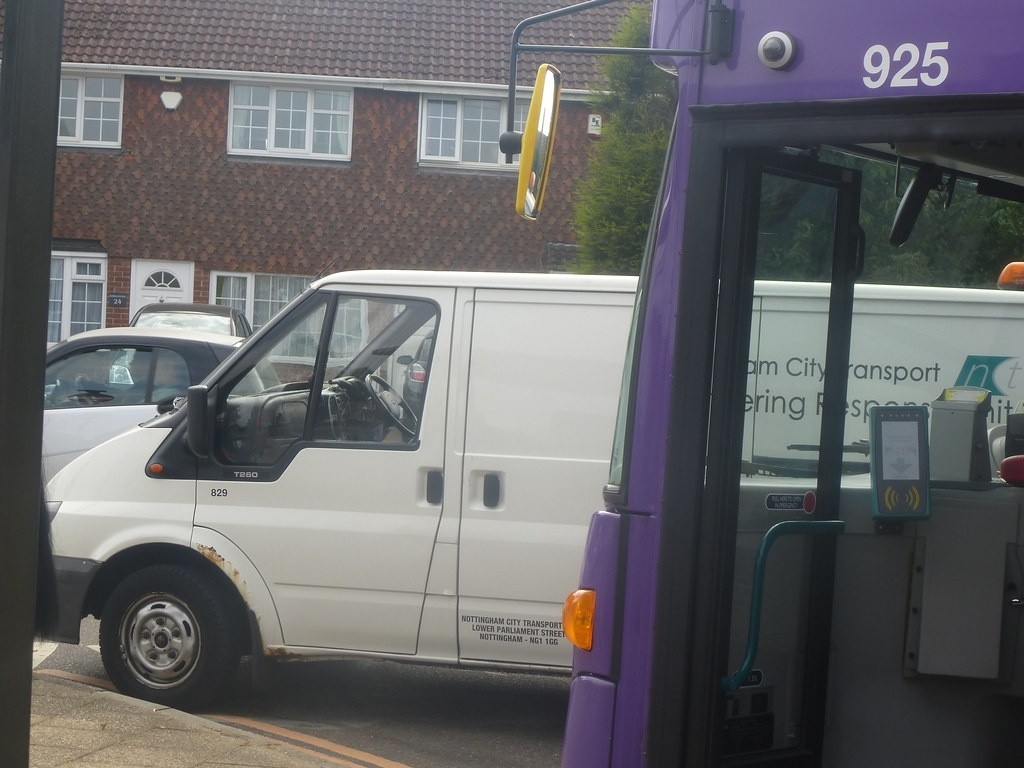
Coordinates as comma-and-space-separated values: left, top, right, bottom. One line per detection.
78, 349, 152, 405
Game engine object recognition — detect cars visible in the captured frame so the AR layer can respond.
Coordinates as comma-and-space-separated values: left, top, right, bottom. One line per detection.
125, 299, 253, 340
41, 325, 282, 488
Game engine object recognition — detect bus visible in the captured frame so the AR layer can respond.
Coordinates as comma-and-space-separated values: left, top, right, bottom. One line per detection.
494, 1, 1024, 768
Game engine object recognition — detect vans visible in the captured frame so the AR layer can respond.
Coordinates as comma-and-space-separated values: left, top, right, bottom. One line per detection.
36, 266, 1024, 714
